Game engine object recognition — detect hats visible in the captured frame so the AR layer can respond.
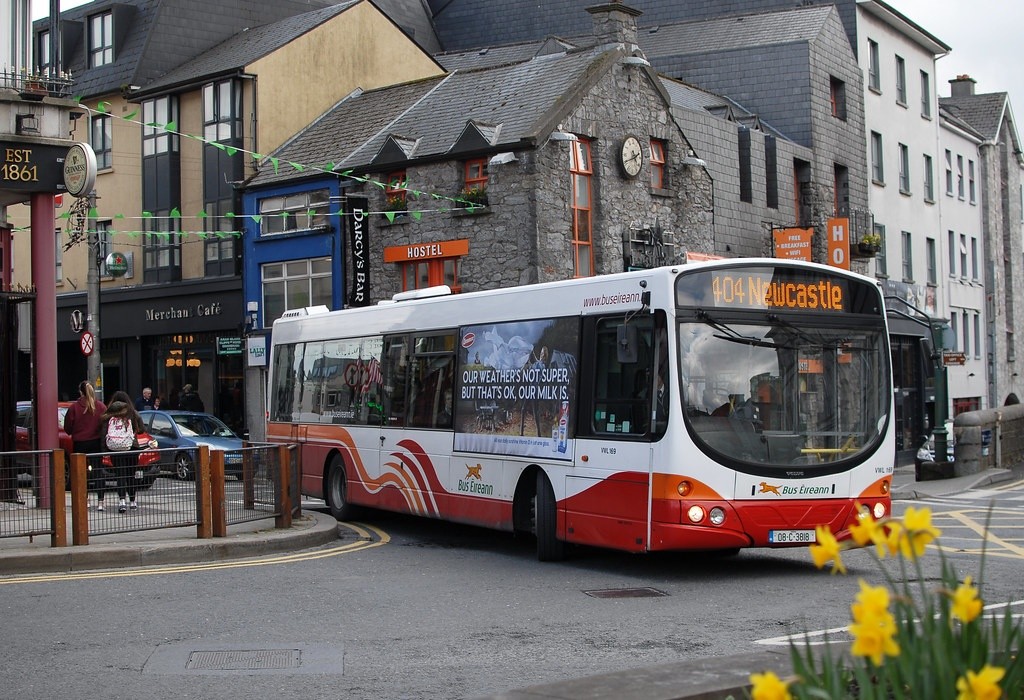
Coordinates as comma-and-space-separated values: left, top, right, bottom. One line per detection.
182, 384, 192, 390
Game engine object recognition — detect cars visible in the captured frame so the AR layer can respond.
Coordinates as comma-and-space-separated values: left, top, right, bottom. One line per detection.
136, 410, 261, 481
915, 418, 955, 481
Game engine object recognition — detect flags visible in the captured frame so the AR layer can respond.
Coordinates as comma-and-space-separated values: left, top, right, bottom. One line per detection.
353, 358, 384, 394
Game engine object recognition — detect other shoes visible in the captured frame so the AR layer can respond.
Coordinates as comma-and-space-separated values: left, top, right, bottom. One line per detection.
97, 504, 107, 511
87, 498, 90, 508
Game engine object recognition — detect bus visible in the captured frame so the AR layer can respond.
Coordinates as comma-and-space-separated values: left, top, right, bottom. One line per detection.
265, 256, 949, 567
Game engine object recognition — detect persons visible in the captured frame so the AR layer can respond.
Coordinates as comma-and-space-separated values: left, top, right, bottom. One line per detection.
65, 380, 110, 513
528, 346, 557, 439
152, 396, 167, 410
178, 383, 206, 412
135, 387, 155, 411
99, 392, 144, 513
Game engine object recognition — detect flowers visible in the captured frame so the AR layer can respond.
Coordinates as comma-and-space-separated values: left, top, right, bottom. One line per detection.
383, 196, 406, 210
861, 234, 880, 246
459, 186, 487, 207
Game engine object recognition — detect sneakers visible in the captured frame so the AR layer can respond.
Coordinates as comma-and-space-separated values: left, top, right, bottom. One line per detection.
130, 505, 137, 510
118, 504, 126, 513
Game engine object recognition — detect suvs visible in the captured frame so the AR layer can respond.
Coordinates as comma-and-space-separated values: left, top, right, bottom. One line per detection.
16, 399, 162, 491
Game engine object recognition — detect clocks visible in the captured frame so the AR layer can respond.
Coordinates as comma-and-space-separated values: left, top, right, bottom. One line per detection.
620, 134, 643, 179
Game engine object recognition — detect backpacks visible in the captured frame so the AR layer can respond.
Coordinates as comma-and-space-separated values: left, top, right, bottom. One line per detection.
106, 416, 134, 451
